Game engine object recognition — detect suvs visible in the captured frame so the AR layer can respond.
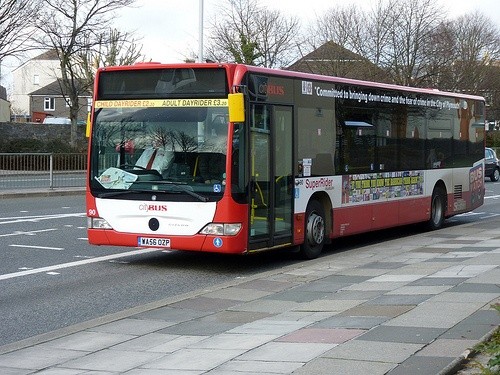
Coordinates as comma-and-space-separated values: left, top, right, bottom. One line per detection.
485, 147, 500, 182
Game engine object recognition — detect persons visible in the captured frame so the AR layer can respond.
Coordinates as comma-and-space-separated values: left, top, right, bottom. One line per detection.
132, 129, 175, 175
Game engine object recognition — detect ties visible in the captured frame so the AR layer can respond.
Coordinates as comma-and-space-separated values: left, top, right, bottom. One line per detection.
146, 148, 159, 170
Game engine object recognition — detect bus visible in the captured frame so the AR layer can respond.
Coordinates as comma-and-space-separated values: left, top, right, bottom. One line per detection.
85, 63, 487, 259
